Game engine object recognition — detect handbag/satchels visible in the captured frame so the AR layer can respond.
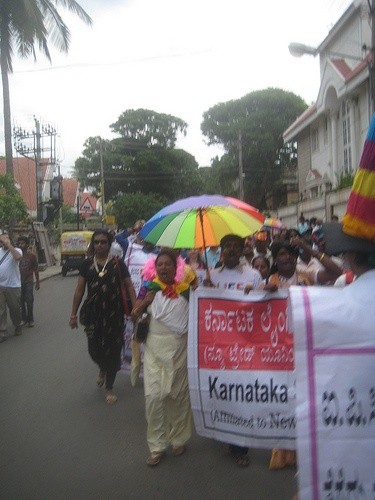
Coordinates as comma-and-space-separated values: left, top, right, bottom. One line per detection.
114, 256, 132, 316
134, 308, 149, 342
131, 335, 139, 387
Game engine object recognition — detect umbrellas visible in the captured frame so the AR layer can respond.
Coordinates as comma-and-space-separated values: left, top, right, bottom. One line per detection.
341, 112, 375, 242
263, 217, 284, 236
142, 194, 266, 287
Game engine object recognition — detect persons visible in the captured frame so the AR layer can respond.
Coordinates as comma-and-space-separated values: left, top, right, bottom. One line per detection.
0, 233, 40, 344
70, 216, 375, 500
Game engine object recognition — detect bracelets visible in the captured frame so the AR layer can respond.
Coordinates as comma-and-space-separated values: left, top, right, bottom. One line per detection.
318, 252, 326, 261
71, 315, 78, 319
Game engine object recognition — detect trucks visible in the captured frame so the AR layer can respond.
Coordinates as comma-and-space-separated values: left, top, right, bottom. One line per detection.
59, 230, 95, 279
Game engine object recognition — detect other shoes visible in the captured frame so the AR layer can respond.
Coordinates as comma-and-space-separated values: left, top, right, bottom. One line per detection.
232, 452, 249, 468
269, 447, 297, 469
96, 373, 106, 387
105, 390, 117, 405
173, 444, 188, 456
0, 337, 7, 343
29, 320, 34, 327
15, 327, 22, 336
146, 452, 166, 466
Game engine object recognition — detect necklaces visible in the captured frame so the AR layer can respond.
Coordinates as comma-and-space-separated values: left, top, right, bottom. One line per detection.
93, 254, 110, 277
